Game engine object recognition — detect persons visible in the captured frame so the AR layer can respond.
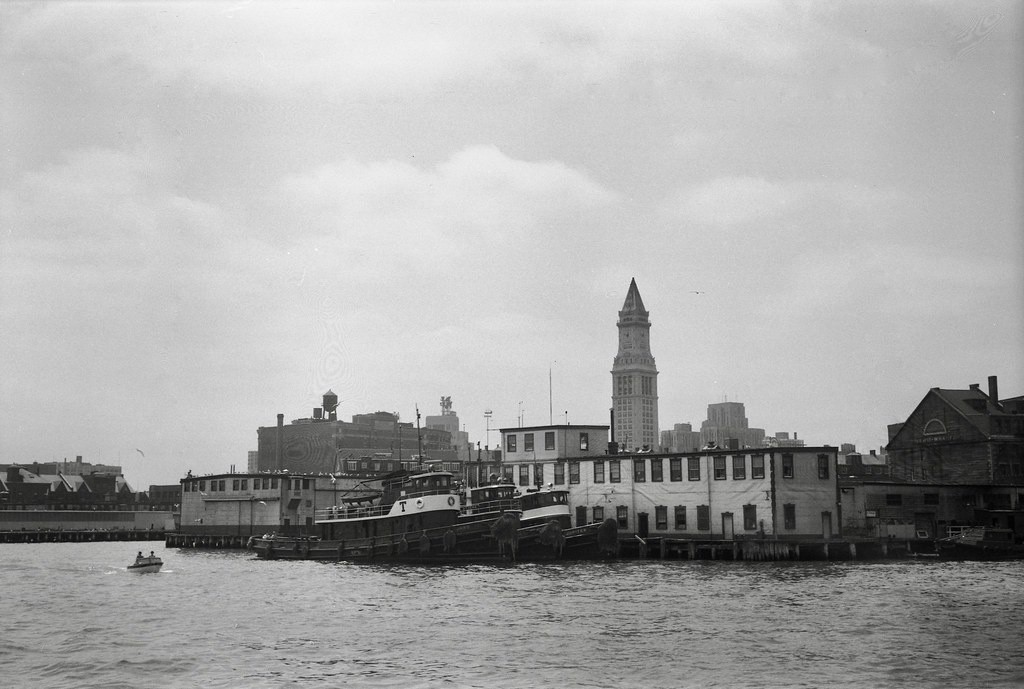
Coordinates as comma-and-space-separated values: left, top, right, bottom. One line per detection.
271, 533, 276, 539
149, 551, 155, 557
263, 533, 270, 539
136, 551, 143, 558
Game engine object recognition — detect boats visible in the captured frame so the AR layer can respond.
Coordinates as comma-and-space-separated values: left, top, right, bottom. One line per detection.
128, 556, 163, 574
937, 529, 1024, 559
248, 410, 617, 565
